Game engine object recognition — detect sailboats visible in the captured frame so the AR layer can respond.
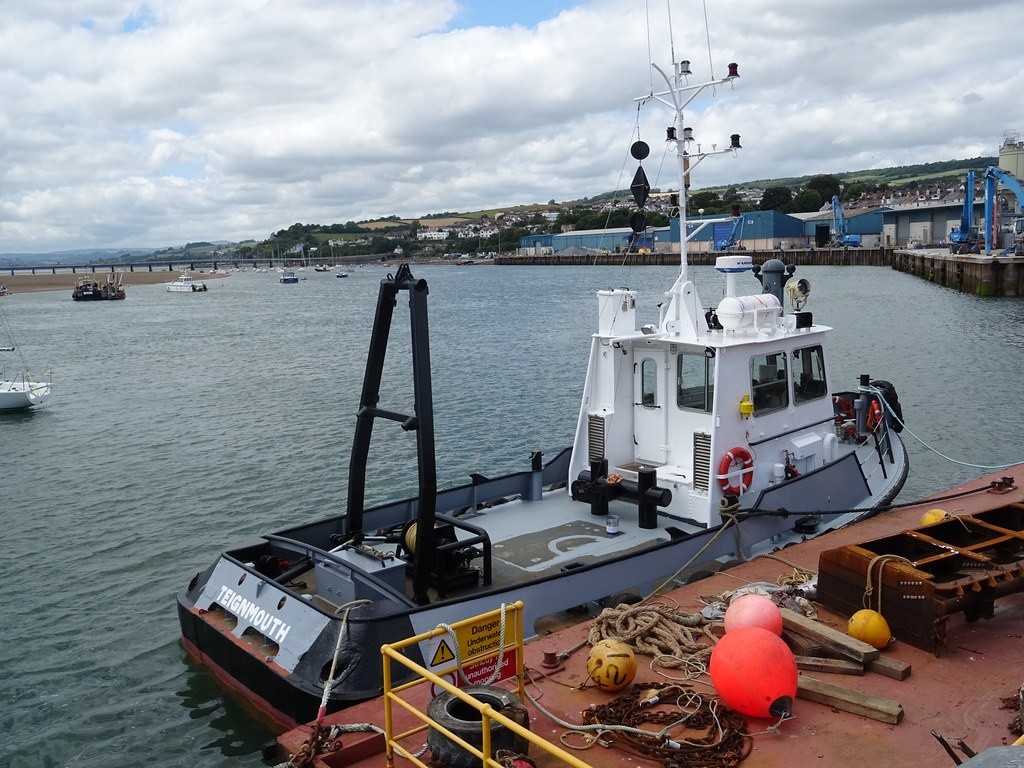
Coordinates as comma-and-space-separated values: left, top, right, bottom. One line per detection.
0, 305, 53, 409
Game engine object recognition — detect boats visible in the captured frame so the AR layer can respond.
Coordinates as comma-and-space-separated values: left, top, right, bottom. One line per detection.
72, 272, 126, 301
173, 2, 910, 730
165, 269, 208, 292
315, 267, 331, 272
279, 271, 298, 283
0, 286, 13, 295
336, 271, 349, 278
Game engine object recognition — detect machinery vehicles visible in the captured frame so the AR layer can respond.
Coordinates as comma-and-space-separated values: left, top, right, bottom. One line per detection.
948, 171, 981, 254
716, 213, 746, 250
983, 165, 1024, 255
832, 195, 861, 247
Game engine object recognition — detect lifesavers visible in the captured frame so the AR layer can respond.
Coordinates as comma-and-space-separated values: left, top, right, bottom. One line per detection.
720, 447, 751, 493
828, 397, 881, 431
